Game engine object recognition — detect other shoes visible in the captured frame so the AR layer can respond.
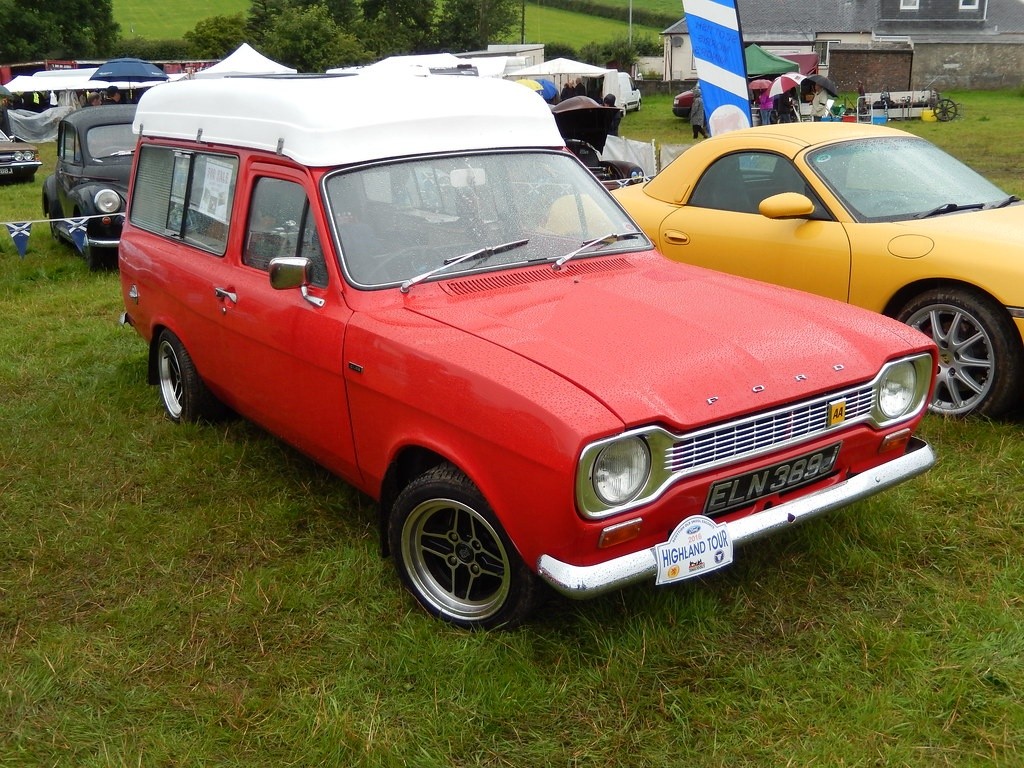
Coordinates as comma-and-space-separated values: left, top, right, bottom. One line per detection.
704, 135, 708, 138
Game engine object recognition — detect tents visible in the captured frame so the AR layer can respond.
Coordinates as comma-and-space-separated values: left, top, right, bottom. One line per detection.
4, 68, 188, 94
502, 58, 621, 106
745, 43, 801, 114
194, 43, 297, 79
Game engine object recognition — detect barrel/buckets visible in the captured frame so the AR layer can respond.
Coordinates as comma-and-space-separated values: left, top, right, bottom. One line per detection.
832, 116, 842, 122
820, 116, 831, 122
842, 115, 856, 122
921, 109, 938, 122
872, 115, 887, 124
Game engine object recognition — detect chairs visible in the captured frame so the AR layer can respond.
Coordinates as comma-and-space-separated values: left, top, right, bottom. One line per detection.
749, 182, 805, 215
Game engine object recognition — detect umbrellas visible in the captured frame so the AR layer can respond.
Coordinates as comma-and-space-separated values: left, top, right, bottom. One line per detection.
89, 57, 170, 99
748, 80, 773, 95
807, 74, 839, 98
0, 85, 13, 96
534, 78, 557, 100
769, 72, 807, 98
516, 79, 543, 91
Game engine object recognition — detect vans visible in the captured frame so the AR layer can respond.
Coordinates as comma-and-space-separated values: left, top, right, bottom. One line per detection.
671, 49, 821, 119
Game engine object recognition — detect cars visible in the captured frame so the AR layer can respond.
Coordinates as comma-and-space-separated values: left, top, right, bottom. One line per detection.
0, 129, 43, 185
551, 95, 647, 191
41, 102, 140, 271
618, 71, 643, 118
544, 121, 1024, 426
110, 71, 941, 637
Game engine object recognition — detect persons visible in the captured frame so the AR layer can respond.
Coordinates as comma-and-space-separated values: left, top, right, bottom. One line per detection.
690, 89, 708, 138
812, 84, 827, 122
778, 89, 791, 122
82, 86, 120, 108
560, 78, 585, 102
603, 94, 620, 136
759, 89, 774, 125
178, 63, 208, 74
0, 96, 11, 137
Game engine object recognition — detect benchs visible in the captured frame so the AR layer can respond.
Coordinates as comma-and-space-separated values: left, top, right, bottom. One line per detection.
864, 88, 932, 118
